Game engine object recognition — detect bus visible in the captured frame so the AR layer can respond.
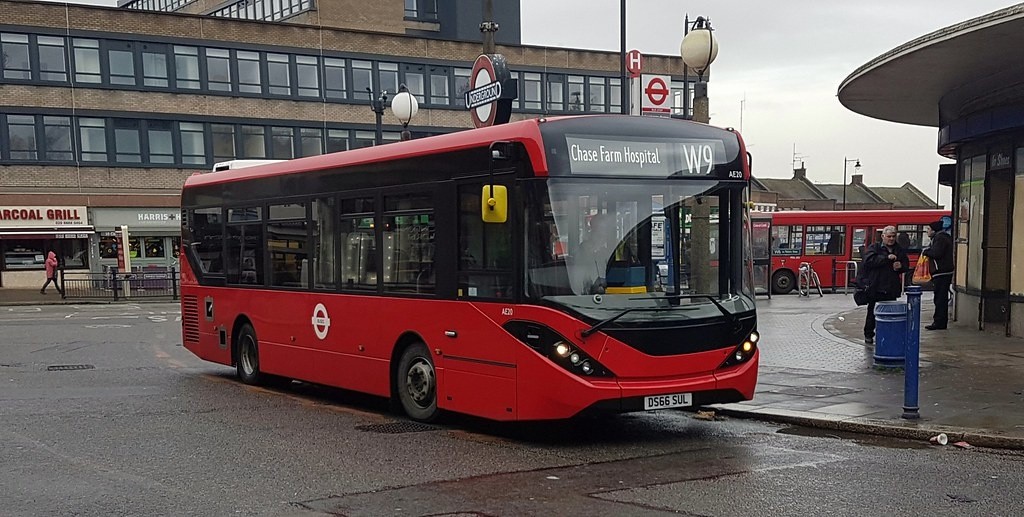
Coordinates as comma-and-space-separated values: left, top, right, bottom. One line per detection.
180, 113, 760, 422
709, 209, 952, 295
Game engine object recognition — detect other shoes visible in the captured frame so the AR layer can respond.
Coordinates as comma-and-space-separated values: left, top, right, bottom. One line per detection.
925, 322, 947, 329
865, 337, 873, 344
40, 291, 48, 294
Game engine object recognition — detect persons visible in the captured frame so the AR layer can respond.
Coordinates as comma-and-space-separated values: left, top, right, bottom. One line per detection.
40, 251, 62, 296
574, 214, 632, 262
923, 220, 955, 330
862, 225, 910, 343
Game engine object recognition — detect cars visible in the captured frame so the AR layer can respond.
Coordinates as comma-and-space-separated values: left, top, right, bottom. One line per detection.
552, 209, 597, 259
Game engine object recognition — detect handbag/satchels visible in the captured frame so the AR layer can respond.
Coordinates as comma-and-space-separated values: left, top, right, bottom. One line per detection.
853, 287, 867, 306
912, 251, 931, 285
53, 267, 58, 279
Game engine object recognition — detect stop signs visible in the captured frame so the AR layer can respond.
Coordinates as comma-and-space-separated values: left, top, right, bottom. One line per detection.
640, 73, 672, 113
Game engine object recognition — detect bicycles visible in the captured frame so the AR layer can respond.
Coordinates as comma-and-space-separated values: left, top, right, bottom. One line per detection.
797, 259, 823, 297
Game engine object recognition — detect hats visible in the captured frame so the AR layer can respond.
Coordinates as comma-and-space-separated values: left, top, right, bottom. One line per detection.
929, 221, 943, 232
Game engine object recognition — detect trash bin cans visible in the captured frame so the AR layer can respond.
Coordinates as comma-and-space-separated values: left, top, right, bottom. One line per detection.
871, 300, 910, 369
121, 275, 131, 298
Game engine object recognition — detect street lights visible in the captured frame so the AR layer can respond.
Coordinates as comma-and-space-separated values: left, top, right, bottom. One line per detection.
842, 157, 861, 210
681, 16, 720, 303
